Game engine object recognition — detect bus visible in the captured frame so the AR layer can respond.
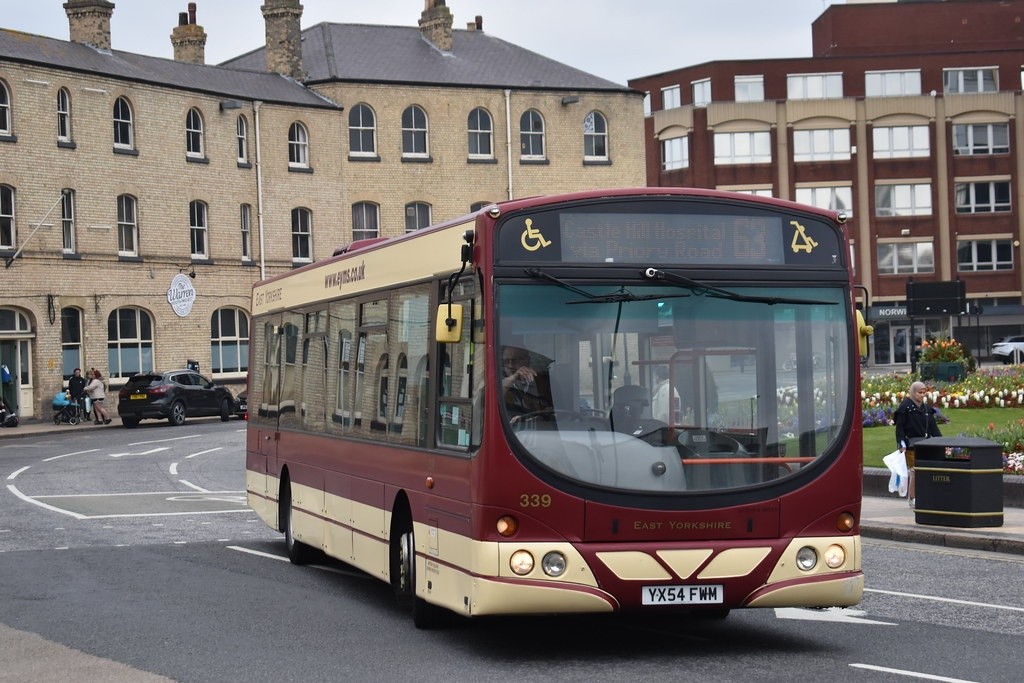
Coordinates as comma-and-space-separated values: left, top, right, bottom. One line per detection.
244, 186, 876, 628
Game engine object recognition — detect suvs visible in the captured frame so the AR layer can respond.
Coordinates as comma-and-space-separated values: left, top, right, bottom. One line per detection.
117, 368, 236, 427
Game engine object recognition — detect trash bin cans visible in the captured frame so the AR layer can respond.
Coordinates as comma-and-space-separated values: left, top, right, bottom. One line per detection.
910, 437, 1004, 529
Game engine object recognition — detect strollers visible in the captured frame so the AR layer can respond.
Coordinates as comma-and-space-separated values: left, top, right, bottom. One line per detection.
52, 389, 87, 425
0, 394, 19, 428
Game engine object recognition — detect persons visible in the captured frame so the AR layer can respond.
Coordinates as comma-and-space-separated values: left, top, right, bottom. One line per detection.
892, 382, 943, 497
502, 346, 556, 422
68, 368, 112, 424
1, 364, 15, 417
652, 365, 681, 426
0, 397, 6, 427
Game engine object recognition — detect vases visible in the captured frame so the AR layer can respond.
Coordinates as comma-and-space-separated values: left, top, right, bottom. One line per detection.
920, 362, 966, 383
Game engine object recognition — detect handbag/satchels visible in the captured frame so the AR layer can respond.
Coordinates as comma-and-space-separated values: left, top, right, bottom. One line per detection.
907, 437, 927, 451
882, 449, 909, 497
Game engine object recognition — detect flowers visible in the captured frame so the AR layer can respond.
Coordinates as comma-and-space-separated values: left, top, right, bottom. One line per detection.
916, 336, 966, 361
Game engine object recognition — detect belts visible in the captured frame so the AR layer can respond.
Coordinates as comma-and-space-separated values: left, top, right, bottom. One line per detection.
92, 398, 104, 401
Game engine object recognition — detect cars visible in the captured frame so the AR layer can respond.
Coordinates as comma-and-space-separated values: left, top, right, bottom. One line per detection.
892, 331, 939, 362
991, 336, 1024, 365
235, 388, 248, 419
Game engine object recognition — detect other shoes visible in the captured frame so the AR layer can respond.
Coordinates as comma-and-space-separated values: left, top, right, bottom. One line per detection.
87, 418, 93, 421
105, 418, 112, 425
94, 421, 103, 425
83, 419, 88, 422
908, 495, 915, 508
